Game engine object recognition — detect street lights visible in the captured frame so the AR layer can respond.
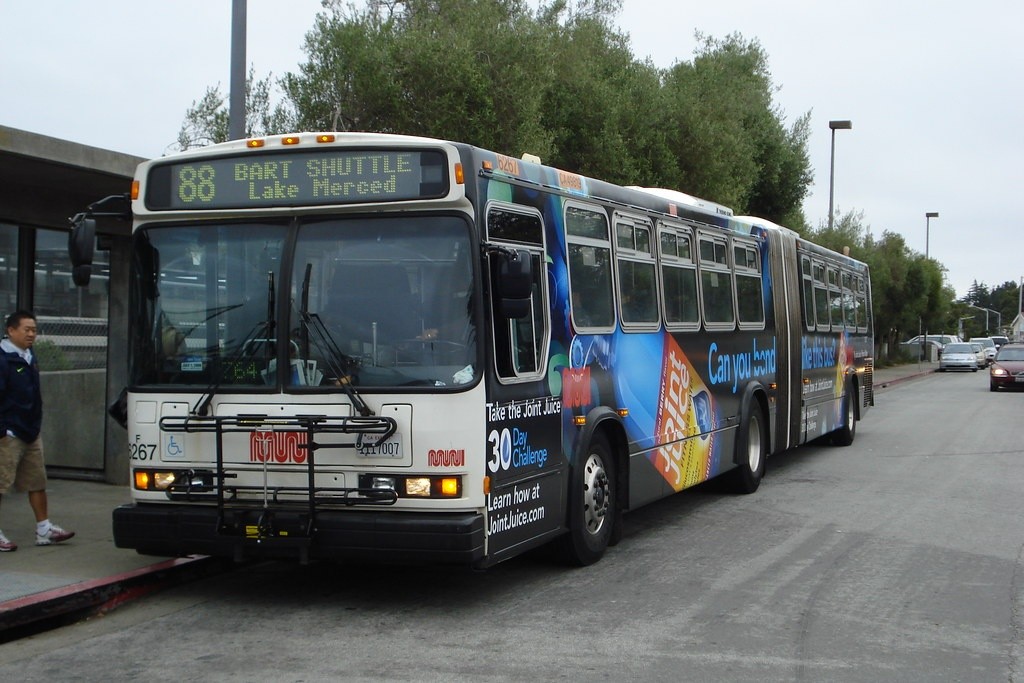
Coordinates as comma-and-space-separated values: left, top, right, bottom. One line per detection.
923, 213, 939, 361
968, 305, 1000, 336
958, 316, 975, 338
829, 120, 852, 250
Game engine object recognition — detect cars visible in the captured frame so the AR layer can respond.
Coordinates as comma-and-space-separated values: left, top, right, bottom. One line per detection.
901, 335, 963, 360
969, 336, 1010, 367
939, 343, 978, 372
970, 342, 988, 370
989, 343, 1024, 391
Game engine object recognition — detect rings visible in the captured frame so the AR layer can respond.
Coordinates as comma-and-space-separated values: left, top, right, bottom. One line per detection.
428, 334, 431, 337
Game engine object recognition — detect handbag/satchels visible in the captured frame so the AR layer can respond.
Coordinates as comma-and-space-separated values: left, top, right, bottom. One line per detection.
108, 386, 127, 431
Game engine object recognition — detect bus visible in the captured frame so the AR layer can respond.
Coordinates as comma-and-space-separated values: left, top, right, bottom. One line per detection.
68, 132, 875, 568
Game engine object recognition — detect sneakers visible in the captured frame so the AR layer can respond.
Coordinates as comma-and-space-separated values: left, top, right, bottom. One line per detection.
34, 524, 75, 546
0, 529, 17, 552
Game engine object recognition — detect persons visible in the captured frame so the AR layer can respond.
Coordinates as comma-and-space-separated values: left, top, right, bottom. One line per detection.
162, 326, 186, 354
416, 281, 469, 340
0, 310, 75, 552
